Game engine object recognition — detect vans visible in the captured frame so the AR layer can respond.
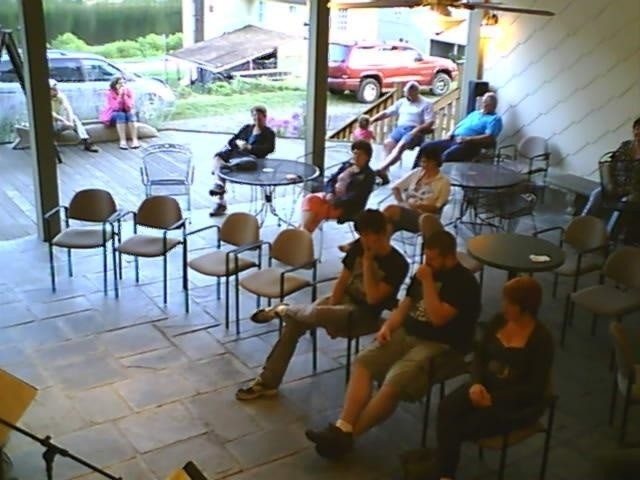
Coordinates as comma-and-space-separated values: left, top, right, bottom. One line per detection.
0, 50, 175, 130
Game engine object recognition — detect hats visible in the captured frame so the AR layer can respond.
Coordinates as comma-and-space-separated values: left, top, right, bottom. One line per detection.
48, 78, 57, 87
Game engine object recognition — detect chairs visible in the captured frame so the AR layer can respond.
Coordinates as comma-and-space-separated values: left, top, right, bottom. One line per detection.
399, 128, 435, 169
308, 276, 400, 385
560, 246, 640, 372
278, 145, 353, 228
376, 181, 470, 280
607, 320, 640, 449
474, 360, 576, 480
417, 211, 485, 291
527, 215, 610, 329
470, 137, 496, 164
497, 136, 552, 205
139, 142, 194, 210
108, 195, 186, 305
598, 150, 626, 211
421, 361, 474, 450
234, 228, 319, 336
302, 171, 378, 263
184, 212, 262, 313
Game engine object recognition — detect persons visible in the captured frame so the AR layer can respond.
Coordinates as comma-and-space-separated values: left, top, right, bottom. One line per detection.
303, 140, 377, 234
412, 277, 554, 480
48, 77, 99, 152
353, 115, 376, 143
338, 144, 451, 253
370, 82, 436, 184
236, 209, 410, 401
579, 117, 640, 243
100, 74, 139, 150
411, 92, 503, 170
209, 106, 276, 217
305, 229, 482, 458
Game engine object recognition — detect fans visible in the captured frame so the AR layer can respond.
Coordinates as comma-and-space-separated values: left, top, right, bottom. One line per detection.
325, 0, 556, 18
42, 189, 123, 296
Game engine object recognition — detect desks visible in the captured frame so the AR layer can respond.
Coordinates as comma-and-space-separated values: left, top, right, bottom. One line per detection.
437, 160, 525, 236
466, 233, 565, 301
216, 157, 320, 231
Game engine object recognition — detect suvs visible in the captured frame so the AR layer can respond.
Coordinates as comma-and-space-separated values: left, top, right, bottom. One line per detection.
327, 39, 458, 104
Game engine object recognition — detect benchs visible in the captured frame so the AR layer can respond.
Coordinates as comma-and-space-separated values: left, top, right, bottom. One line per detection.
548, 174, 601, 214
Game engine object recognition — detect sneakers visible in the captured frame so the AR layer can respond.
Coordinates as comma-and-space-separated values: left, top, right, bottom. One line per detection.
305, 424, 356, 453
120, 142, 129, 149
131, 141, 140, 148
210, 181, 227, 195
209, 201, 229, 218
236, 377, 279, 404
85, 139, 98, 152
315, 441, 343, 462
252, 303, 289, 328
